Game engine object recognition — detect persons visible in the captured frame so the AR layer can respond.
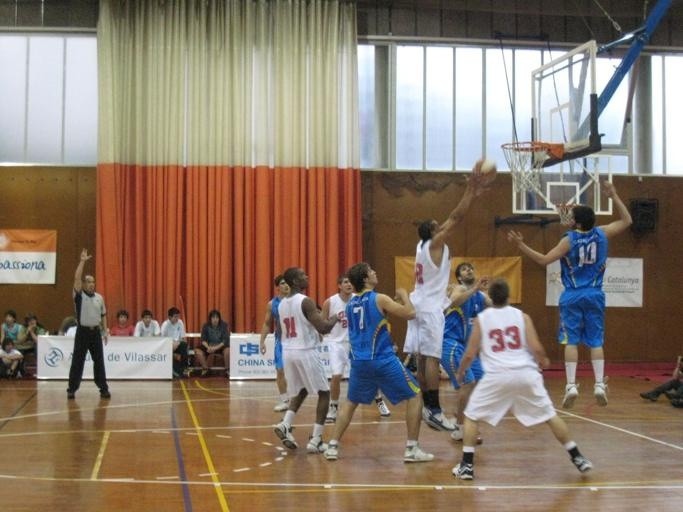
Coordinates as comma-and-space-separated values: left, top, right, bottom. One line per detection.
0, 308, 188, 379
507, 180, 633, 408
194, 311, 230, 378
403, 167, 492, 431
323, 262, 435, 464
438, 262, 494, 445
639, 355, 683, 408
451, 281, 592, 477
322, 272, 390, 423
258, 273, 291, 413
275, 265, 341, 453
66, 248, 110, 400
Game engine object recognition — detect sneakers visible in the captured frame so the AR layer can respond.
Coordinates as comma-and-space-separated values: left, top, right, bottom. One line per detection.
275, 422, 298, 450
640, 390, 683, 407
451, 426, 483, 445
404, 446, 434, 462
594, 383, 609, 407
422, 407, 460, 432
307, 434, 328, 452
101, 391, 110, 398
324, 404, 338, 423
273, 399, 291, 412
562, 384, 579, 409
376, 401, 391, 416
574, 457, 593, 472
452, 462, 473, 480
200, 368, 210, 377
68, 393, 74, 399
324, 444, 338, 459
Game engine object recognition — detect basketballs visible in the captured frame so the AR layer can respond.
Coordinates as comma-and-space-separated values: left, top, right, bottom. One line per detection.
473, 160, 496, 186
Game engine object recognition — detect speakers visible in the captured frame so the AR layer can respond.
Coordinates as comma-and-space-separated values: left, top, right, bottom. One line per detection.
632, 200, 657, 235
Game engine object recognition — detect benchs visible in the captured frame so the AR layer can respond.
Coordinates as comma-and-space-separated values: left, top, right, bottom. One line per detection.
183, 331, 232, 379
